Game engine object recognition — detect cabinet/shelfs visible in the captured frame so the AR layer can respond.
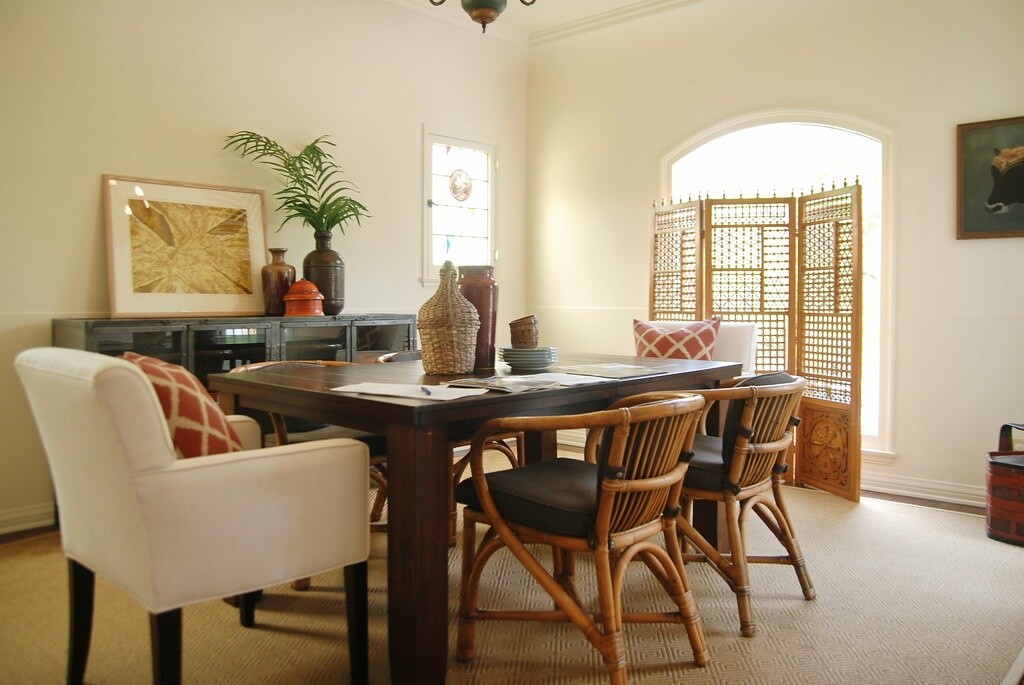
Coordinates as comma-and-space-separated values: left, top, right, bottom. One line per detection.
50, 313, 418, 532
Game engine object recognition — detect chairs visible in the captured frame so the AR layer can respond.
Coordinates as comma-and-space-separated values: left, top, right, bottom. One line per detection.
454, 391, 711, 685
14, 346, 371, 685
649, 321, 758, 438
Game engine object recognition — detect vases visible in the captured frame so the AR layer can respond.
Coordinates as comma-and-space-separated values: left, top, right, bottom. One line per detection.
456, 265, 499, 369
261, 247, 296, 317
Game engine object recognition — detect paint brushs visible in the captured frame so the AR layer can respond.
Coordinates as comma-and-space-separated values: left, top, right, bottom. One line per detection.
421, 386, 432, 396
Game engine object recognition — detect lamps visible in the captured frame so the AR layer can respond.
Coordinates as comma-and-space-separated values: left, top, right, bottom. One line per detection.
429, 0, 536, 34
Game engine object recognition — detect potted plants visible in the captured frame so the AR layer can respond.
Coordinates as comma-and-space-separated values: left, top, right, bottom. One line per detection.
223, 130, 373, 315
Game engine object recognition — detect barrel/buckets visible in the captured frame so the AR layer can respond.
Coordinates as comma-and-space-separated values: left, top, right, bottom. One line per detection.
985, 423, 1024, 542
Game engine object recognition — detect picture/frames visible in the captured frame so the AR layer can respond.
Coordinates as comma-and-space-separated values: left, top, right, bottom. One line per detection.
102, 173, 272, 319
955, 116, 1024, 240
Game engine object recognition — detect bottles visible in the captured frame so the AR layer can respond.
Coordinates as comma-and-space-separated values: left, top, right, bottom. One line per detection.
261, 248, 296, 317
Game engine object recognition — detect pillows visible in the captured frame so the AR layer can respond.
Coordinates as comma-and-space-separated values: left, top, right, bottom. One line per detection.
118, 351, 244, 459
722, 371, 796, 466
633, 316, 721, 361
595, 398, 683, 531
584, 374, 817, 638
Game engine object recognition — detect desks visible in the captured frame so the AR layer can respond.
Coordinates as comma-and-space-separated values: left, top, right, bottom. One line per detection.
207, 353, 743, 685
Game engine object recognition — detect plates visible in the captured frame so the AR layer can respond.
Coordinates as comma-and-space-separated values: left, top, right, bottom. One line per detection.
498, 348, 557, 372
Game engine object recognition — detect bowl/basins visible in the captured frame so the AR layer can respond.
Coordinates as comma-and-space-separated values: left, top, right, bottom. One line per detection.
509, 314, 539, 349
